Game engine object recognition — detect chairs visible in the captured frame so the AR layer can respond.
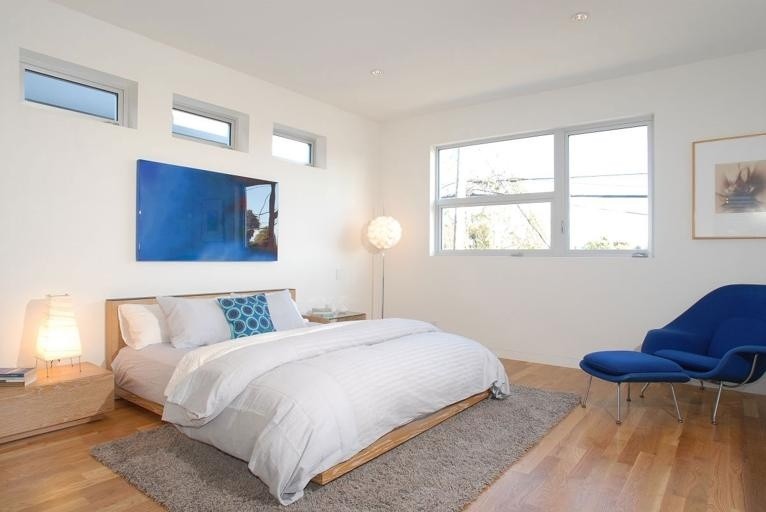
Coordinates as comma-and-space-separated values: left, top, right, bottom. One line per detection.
638, 283, 766, 424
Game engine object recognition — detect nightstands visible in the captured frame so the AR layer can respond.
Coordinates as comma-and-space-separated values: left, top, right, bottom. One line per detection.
0, 361, 117, 443
303, 308, 367, 324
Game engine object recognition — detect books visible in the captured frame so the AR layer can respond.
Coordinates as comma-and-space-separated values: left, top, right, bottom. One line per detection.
0, 367, 36, 387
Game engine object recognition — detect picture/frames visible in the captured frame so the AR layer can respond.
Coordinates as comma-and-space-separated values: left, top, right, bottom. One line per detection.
691, 132, 766, 240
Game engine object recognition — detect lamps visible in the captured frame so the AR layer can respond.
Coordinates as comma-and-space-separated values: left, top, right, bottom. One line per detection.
33, 291, 83, 378
366, 215, 403, 318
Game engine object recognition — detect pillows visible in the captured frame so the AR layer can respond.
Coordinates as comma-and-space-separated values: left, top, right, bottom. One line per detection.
117, 288, 310, 351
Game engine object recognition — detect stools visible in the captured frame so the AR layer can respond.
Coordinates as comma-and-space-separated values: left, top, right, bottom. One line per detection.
580, 349, 691, 427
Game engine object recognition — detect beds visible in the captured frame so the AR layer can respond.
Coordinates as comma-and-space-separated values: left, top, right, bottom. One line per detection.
103, 288, 500, 486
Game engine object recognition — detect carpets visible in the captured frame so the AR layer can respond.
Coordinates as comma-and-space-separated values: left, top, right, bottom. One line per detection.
86, 379, 582, 512
